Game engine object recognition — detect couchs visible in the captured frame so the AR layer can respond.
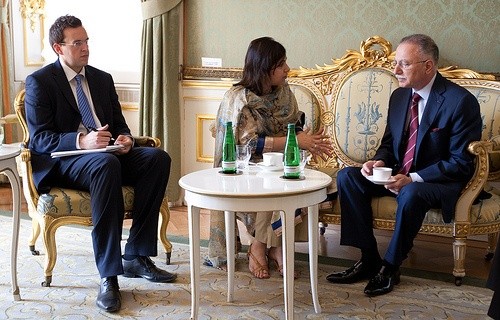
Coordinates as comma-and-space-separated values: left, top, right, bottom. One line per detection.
277, 37, 500, 282
13, 88, 175, 288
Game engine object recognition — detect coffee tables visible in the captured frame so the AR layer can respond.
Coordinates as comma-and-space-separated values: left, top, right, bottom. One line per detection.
177, 163, 332, 320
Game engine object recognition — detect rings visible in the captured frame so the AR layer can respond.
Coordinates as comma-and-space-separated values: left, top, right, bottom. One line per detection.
314, 144, 318, 148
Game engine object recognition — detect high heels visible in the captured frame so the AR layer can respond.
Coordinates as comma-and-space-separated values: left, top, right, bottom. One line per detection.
246, 244, 271, 278
267, 246, 299, 280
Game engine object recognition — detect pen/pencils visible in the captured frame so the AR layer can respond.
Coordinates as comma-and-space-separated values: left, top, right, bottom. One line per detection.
87, 126, 114, 140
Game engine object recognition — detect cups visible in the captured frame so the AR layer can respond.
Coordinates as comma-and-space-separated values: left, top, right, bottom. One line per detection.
373, 167, 392, 181
236, 145, 251, 174
262, 152, 283, 167
299, 150, 306, 179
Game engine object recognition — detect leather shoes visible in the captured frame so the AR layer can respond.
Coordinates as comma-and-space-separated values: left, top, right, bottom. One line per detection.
96, 275, 122, 312
363, 259, 400, 297
325, 260, 383, 284
121, 255, 177, 283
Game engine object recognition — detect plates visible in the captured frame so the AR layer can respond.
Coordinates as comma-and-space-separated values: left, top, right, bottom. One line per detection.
256, 162, 284, 171
366, 175, 397, 185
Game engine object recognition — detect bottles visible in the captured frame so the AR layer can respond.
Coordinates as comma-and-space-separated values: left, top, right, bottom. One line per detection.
222, 121, 236, 174
283, 124, 299, 179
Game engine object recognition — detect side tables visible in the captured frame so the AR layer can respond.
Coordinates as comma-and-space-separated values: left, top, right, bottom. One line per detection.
0, 144, 22, 302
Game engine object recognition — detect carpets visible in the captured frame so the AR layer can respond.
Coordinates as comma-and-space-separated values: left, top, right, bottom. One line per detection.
0, 212, 500, 320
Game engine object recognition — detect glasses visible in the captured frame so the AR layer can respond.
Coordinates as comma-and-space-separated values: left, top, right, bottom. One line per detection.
58, 39, 88, 47
393, 59, 431, 69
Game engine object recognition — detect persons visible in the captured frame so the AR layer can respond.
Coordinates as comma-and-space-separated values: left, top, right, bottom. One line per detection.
207, 37, 334, 283
324, 35, 483, 295
26, 16, 178, 312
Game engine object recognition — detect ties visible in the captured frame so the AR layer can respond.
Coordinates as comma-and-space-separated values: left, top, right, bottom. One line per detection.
75, 75, 96, 129
398, 92, 422, 175
